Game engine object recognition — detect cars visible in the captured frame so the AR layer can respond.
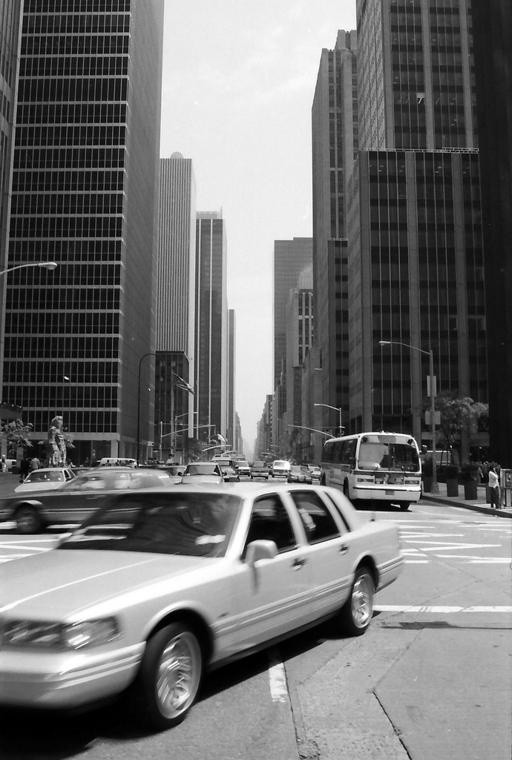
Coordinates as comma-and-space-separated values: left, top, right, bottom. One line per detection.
0, 477, 407, 732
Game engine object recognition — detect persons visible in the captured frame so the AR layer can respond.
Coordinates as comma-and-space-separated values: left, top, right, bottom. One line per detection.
154, 458, 157, 464
28, 455, 41, 479
488, 466, 501, 509
195, 499, 231, 551
19, 457, 30, 482
477, 467, 484, 483
65, 458, 76, 468
165, 454, 174, 464
99, 459, 137, 467
84, 457, 89, 467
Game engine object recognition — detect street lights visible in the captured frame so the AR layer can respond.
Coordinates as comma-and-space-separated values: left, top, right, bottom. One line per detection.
285, 423, 338, 439
0, 260, 59, 275
313, 403, 344, 436
173, 410, 199, 462
378, 339, 440, 495
136, 352, 165, 472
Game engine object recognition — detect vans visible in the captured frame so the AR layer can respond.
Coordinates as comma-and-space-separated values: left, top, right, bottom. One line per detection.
419, 448, 450, 465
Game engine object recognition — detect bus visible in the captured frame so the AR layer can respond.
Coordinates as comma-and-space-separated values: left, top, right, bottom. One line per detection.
0, 467, 175, 538
320, 432, 423, 510
